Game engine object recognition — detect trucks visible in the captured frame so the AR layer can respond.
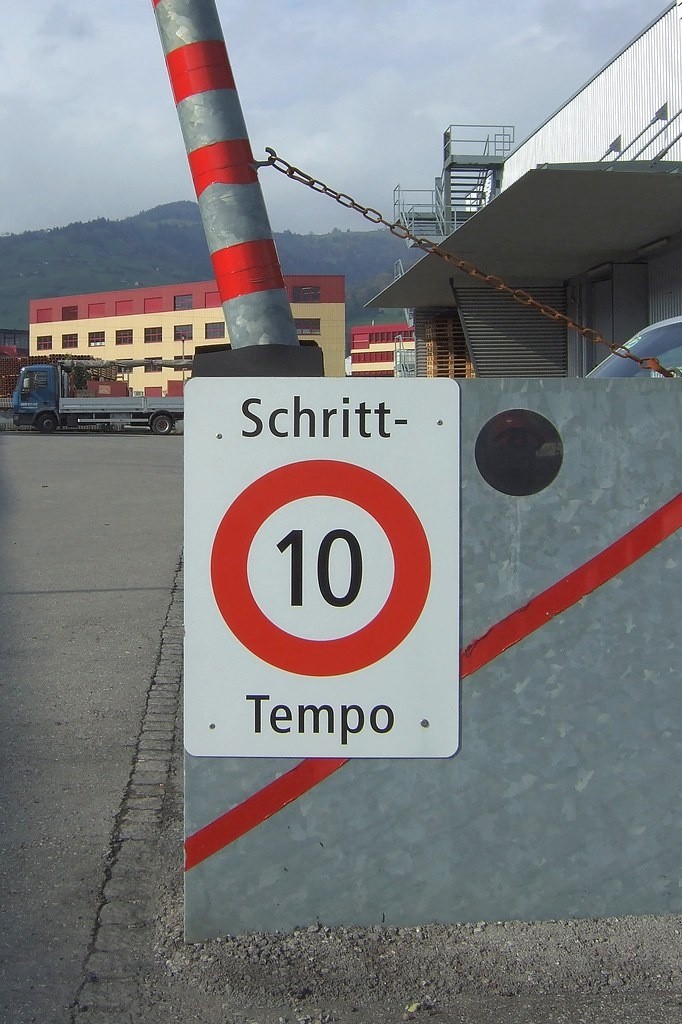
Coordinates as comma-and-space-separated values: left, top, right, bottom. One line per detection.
13, 364, 185, 437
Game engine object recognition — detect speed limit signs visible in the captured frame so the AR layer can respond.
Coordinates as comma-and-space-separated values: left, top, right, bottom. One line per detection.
184, 373, 460, 759
